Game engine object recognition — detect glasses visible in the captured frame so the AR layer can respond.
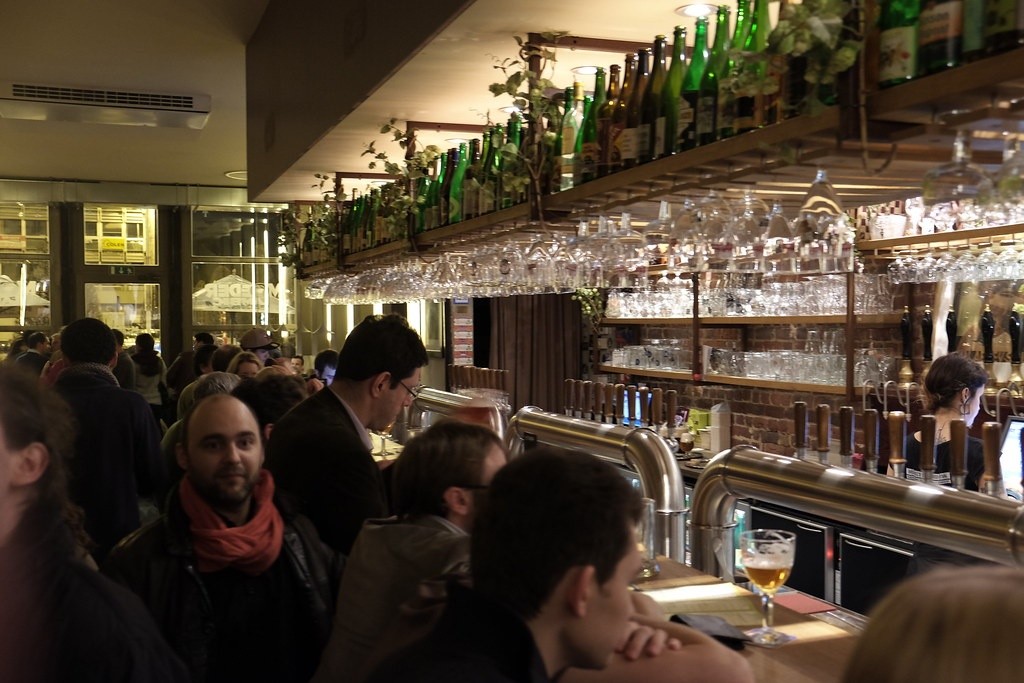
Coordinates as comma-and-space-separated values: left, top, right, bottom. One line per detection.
381, 371, 418, 402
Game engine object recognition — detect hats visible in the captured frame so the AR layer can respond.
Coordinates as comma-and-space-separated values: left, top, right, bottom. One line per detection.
240, 328, 281, 351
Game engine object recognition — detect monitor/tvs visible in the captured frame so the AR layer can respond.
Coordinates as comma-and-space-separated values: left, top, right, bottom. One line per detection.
623, 388, 652, 428
999, 415, 1024, 502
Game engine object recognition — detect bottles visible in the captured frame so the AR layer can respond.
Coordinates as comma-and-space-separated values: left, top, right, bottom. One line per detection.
297, 1, 776, 266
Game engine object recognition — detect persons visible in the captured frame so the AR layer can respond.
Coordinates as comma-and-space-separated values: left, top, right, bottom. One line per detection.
101, 392, 336, 683
310, 418, 755, 683
262, 316, 428, 551
39, 317, 161, 566
860, 354, 1007, 499
840, 564, 1024, 683
0, 362, 192, 683
8, 328, 339, 448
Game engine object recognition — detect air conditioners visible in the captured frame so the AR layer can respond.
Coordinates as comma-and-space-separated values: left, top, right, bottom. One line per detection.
0, 78, 212, 130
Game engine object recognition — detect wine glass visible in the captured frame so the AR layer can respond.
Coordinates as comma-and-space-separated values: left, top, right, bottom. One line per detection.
738, 529, 797, 648
304, 160, 1024, 389
